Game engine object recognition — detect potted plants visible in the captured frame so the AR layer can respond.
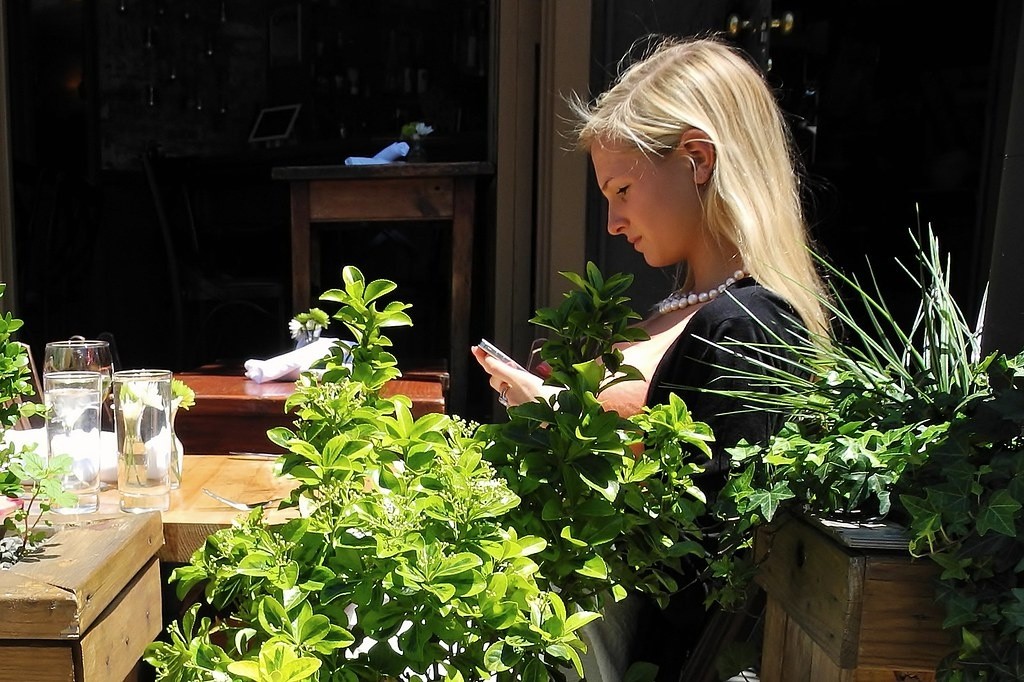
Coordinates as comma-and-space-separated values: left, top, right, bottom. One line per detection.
1, 441, 79, 571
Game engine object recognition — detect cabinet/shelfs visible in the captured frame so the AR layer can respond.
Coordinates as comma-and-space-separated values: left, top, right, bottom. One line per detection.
0, 511, 166, 682
750, 504, 966, 682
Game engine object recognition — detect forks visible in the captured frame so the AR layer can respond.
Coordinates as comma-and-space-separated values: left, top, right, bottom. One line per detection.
203, 489, 284, 512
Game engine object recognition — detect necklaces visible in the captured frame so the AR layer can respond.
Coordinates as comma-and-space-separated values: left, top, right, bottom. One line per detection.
658, 263, 751, 315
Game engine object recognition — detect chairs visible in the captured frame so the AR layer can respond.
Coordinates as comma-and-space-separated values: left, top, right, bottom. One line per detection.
145, 154, 286, 371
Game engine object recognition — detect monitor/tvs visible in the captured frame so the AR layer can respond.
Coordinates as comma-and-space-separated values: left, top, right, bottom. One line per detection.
248, 104, 302, 150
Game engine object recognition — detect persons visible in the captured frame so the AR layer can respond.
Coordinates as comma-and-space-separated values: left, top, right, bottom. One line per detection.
470, 39, 844, 682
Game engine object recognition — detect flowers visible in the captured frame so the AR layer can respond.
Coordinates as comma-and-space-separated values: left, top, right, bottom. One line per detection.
156, 378, 195, 484
288, 307, 331, 343
109, 373, 163, 487
119, 377, 196, 488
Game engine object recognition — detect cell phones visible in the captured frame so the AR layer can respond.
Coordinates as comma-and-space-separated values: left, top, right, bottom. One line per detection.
477, 338, 529, 374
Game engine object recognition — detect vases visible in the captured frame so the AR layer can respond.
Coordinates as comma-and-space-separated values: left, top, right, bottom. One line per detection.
163, 406, 183, 488
111, 408, 149, 498
403, 136, 430, 163
118, 406, 185, 498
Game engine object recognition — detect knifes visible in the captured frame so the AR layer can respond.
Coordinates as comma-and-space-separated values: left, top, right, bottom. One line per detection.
230, 450, 283, 457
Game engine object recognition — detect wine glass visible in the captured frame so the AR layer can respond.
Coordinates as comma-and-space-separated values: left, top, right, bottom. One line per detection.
45, 341, 110, 436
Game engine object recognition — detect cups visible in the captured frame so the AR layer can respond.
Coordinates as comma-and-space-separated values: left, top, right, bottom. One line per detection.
43, 371, 103, 514
114, 369, 170, 513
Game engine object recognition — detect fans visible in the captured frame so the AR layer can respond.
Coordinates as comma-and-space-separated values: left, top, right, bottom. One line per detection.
400, 121, 434, 139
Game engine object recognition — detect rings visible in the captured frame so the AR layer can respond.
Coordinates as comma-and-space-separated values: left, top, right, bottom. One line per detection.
498, 385, 510, 406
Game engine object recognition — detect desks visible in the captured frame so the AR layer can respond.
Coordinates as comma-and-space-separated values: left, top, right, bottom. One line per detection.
22, 454, 288, 562
171, 370, 453, 456
270, 160, 494, 423
